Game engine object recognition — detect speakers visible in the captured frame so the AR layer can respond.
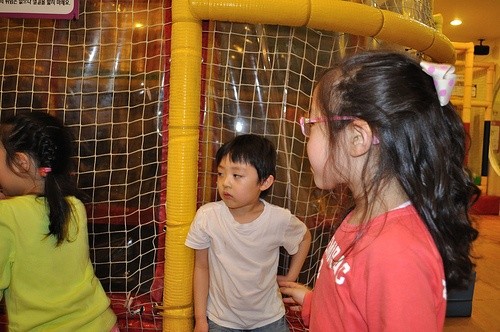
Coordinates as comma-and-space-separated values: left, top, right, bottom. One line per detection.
474, 45, 490, 56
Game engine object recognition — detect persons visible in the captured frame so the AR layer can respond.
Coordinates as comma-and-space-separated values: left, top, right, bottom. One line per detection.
278, 51, 481, 332
0, 112, 119, 332
185, 134, 311, 332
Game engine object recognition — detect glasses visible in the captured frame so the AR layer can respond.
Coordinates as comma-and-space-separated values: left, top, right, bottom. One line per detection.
299, 116, 380, 145
